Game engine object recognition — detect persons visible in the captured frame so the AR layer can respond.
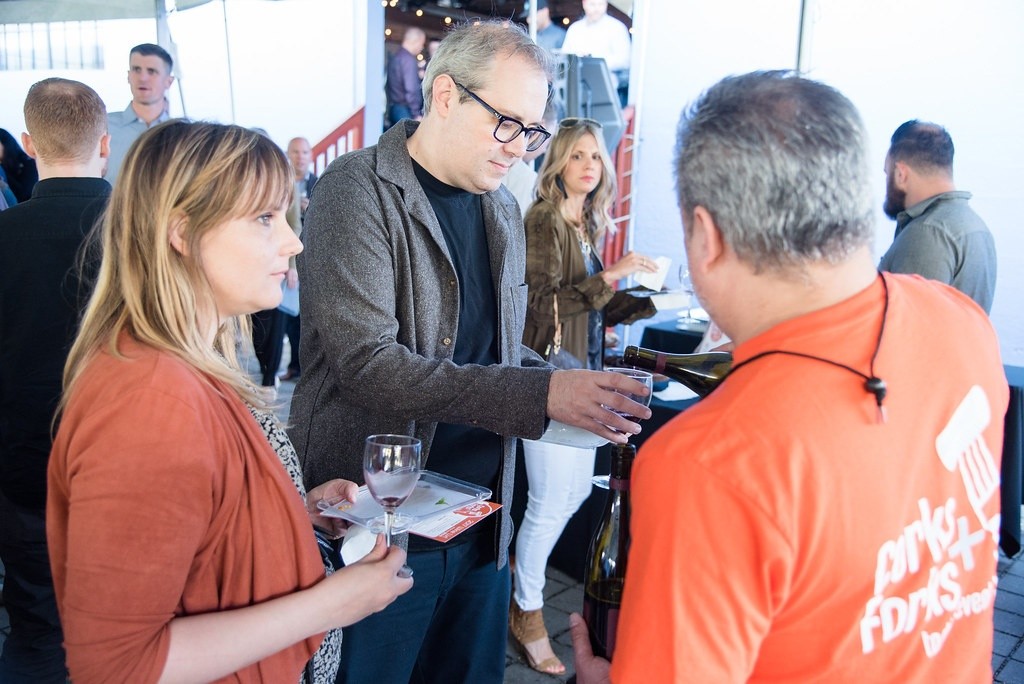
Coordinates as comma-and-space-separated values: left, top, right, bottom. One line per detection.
518, 0, 565, 54
101, 43, 175, 191
428, 38, 442, 56
570, 72, 1010, 684
279, 138, 318, 385
387, 28, 423, 124
286, 23, 653, 684
0, 129, 39, 211
0, 79, 114, 684
250, 190, 298, 390
510, 119, 657, 674
562, 0, 631, 109
45, 118, 415, 684
877, 120, 997, 316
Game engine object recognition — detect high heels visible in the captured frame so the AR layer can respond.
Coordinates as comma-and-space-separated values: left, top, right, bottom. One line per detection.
508, 600, 568, 677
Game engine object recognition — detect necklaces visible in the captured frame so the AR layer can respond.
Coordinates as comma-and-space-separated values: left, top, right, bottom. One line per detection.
576, 228, 591, 255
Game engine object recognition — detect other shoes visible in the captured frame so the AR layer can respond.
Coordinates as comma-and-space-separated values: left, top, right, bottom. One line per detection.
278, 368, 302, 380
274, 376, 280, 388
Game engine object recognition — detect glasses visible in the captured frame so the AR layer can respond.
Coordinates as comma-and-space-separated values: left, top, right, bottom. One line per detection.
456, 81, 551, 152
554, 118, 604, 137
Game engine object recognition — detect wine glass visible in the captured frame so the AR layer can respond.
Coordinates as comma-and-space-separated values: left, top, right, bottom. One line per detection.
582, 443, 637, 664
590, 367, 653, 490
362, 433, 422, 579
676, 262, 701, 324
298, 190, 307, 219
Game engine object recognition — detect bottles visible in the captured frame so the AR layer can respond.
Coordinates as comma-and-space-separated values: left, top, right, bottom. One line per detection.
624, 345, 734, 399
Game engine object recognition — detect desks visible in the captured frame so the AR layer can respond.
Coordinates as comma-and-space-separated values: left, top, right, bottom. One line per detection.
641, 317, 708, 356
998, 364, 1024, 558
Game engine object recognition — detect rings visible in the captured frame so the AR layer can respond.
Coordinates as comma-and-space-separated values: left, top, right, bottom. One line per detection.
642, 259, 646, 266
